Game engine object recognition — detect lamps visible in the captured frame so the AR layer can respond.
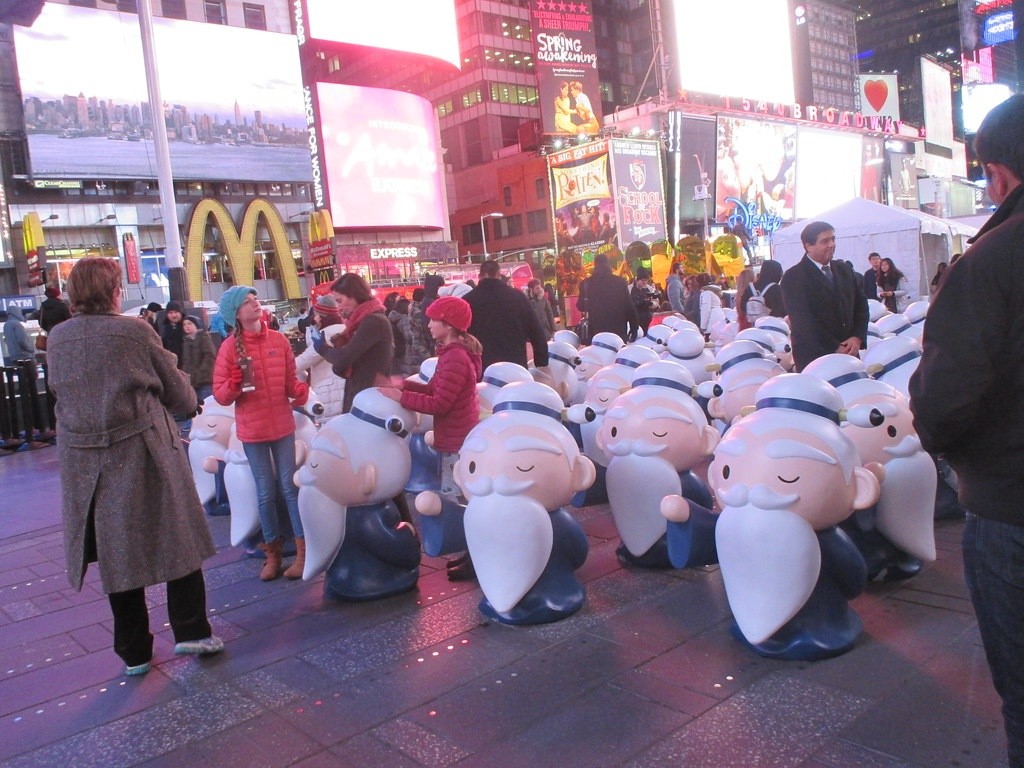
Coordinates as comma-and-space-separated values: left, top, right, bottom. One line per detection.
42, 215, 58, 223
564, 138, 571, 148
290, 211, 310, 218
100, 215, 116, 221
539, 146, 546, 155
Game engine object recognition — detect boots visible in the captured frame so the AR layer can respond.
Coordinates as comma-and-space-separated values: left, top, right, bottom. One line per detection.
259, 536, 283, 581
283, 538, 305, 577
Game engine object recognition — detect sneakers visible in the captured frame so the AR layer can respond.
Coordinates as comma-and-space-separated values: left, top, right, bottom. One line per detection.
447, 558, 472, 580
126, 647, 154, 675
446, 551, 472, 568
174, 635, 224, 653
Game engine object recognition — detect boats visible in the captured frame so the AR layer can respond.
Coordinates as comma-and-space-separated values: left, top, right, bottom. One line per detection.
57, 127, 312, 149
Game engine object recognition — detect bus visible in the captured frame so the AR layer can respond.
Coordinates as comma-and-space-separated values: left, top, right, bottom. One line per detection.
310, 259, 535, 322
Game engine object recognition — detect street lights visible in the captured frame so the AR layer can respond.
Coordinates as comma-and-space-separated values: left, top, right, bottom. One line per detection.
480, 213, 504, 262
692, 153, 708, 240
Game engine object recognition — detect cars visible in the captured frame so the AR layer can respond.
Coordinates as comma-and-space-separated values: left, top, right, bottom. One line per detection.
0, 320, 47, 383
257, 298, 303, 334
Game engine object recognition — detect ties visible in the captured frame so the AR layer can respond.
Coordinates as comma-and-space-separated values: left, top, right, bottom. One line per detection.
822, 265, 835, 288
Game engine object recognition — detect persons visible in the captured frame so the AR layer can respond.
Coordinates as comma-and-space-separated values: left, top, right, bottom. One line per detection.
554, 81, 579, 133
734, 221, 909, 376
576, 255, 641, 345
570, 81, 599, 133
140, 301, 217, 430
556, 206, 617, 245
905, 93, 1024, 766
378, 298, 484, 582
3, 306, 38, 395
379, 272, 473, 377
462, 260, 550, 380
212, 284, 309, 580
659, 298, 960, 660
623, 261, 727, 341
311, 272, 395, 414
37, 285, 72, 333
900, 158, 914, 207
861, 142, 881, 203
503, 276, 561, 342
293, 294, 347, 419
43, 255, 224, 675
411, 313, 720, 623
186, 355, 467, 602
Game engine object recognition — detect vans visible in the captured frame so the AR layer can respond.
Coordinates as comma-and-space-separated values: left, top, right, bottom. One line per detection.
123, 300, 221, 319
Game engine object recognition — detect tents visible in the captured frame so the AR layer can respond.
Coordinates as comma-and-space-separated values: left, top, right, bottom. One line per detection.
770, 197, 980, 314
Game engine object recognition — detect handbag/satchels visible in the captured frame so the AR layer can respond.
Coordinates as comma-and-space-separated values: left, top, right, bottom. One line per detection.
573, 279, 590, 344
36, 335, 47, 351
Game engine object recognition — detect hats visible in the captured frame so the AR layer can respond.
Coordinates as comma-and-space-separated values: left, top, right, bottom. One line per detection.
314, 294, 339, 315
45, 285, 60, 297
185, 315, 201, 330
423, 273, 444, 296
637, 270, 649, 280
165, 301, 183, 314
437, 284, 473, 300
218, 285, 258, 330
425, 296, 472, 332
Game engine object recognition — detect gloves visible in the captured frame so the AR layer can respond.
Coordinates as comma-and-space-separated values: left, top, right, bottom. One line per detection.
230, 366, 244, 392
289, 384, 308, 407
627, 331, 638, 342
310, 329, 325, 352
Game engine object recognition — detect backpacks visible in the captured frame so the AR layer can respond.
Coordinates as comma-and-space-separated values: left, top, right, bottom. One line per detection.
746, 282, 776, 324
389, 318, 405, 359
